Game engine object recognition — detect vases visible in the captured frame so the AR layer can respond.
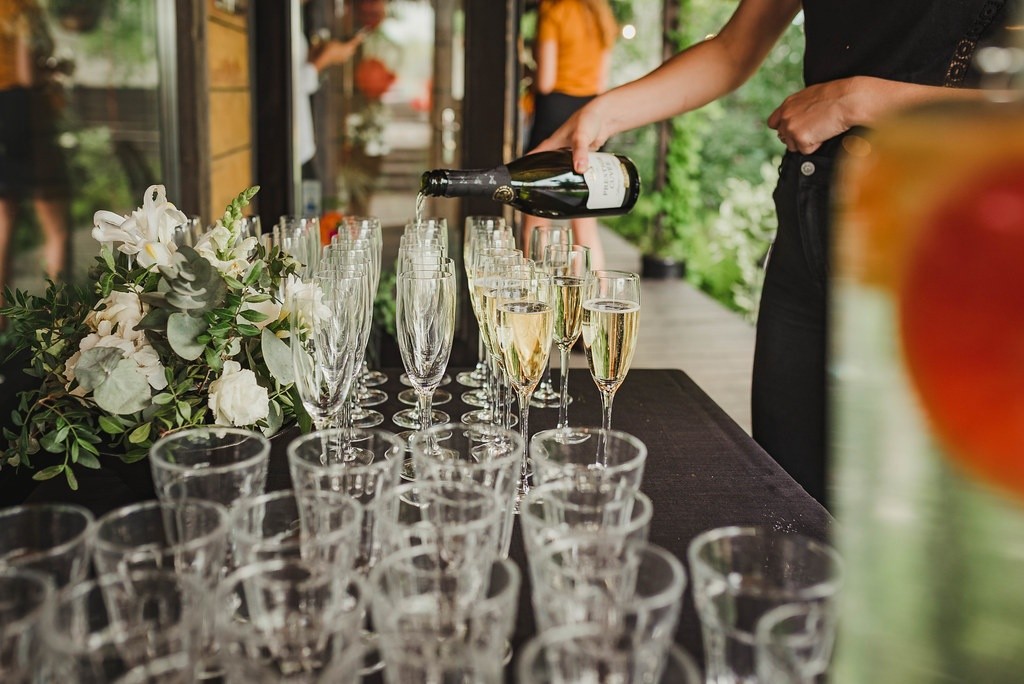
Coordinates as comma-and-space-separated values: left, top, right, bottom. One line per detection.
143, 400, 284, 439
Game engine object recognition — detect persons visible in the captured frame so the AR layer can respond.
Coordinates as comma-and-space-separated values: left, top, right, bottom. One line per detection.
519, 0, 616, 298
291, 0, 365, 180
0, 0, 79, 301
527, 0, 1024, 514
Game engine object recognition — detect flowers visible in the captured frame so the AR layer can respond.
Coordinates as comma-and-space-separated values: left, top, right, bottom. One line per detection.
0, 183, 334, 492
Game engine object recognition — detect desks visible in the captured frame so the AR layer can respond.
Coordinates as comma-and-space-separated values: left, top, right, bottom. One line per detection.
0, 367, 836, 683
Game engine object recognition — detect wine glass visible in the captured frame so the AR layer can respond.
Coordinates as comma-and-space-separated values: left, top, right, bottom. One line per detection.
0, 211, 842, 684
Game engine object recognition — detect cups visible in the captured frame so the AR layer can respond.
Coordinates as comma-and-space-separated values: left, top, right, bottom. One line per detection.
831, 96, 1024, 683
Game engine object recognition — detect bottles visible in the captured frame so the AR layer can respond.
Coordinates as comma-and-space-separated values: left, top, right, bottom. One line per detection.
421, 149, 641, 220
